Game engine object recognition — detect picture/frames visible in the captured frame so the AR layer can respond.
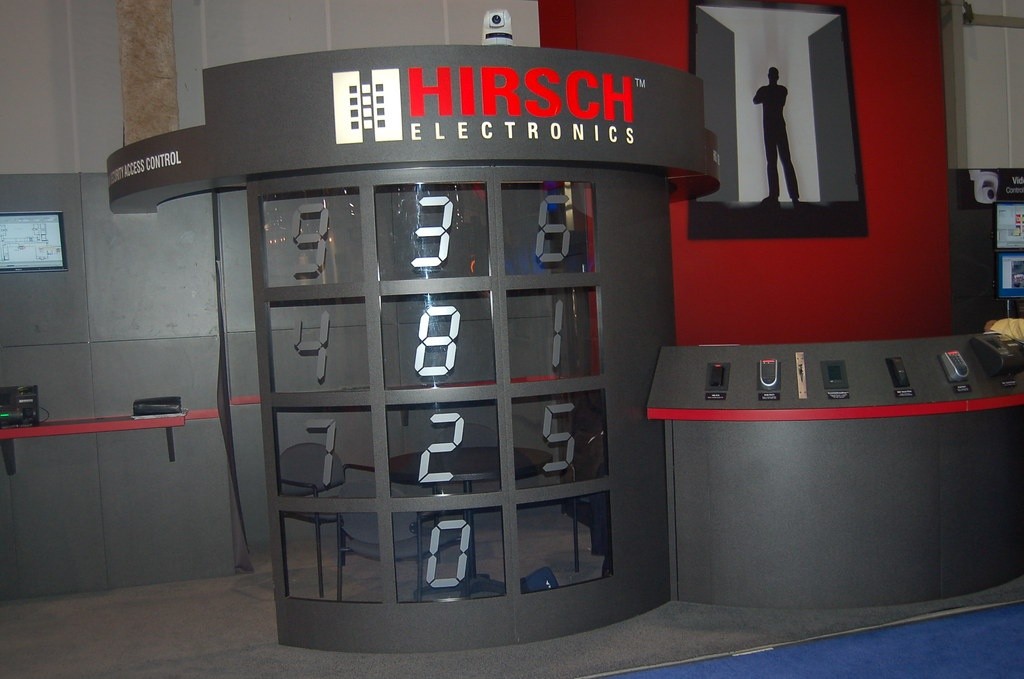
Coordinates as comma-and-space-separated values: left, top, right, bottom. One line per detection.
0, 210, 69, 274
687, 2, 867, 240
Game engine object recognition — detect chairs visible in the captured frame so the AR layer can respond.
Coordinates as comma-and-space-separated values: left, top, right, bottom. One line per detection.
335, 478, 470, 601
279, 443, 375, 597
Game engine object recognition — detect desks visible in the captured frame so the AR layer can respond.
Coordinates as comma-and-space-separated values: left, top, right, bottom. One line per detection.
390, 447, 554, 600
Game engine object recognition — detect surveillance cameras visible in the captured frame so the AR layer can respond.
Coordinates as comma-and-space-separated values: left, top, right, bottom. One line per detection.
482, 9, 514, 46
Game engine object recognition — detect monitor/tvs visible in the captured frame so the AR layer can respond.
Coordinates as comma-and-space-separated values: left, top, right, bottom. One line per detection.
0, 211, 69, 272
994, 200, 1024, 300
